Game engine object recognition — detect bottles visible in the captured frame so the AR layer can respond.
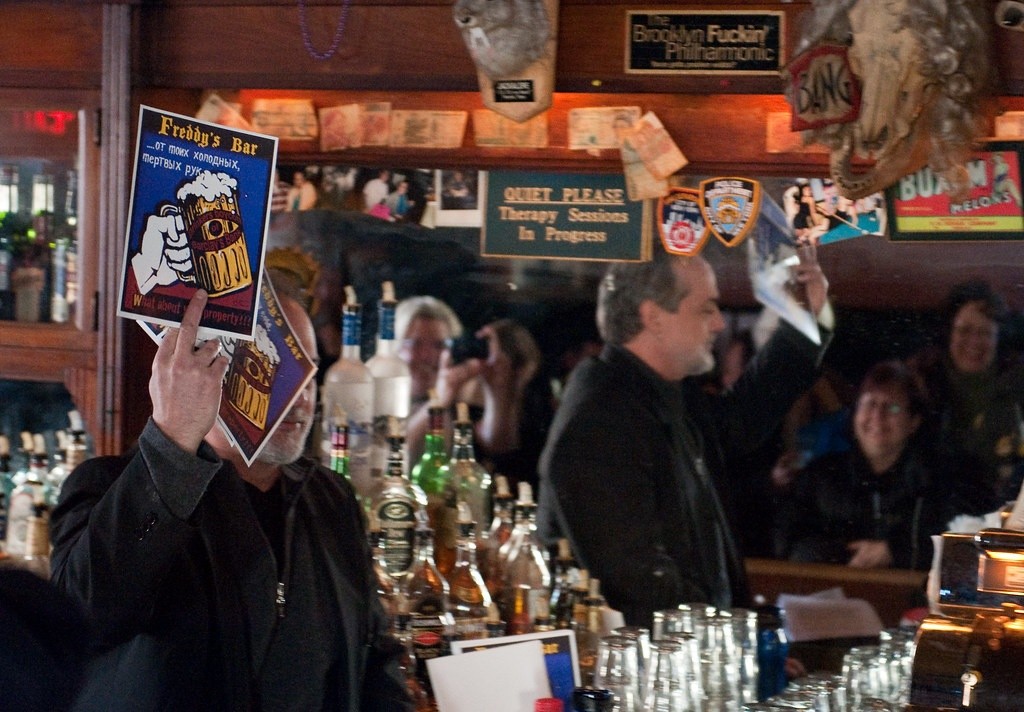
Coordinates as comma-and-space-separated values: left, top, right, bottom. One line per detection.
363, 281, 411, 480
328, 401, 627, 711
0, 430, 94, 581
321, 286, 374, 489
0, 211, 79, 325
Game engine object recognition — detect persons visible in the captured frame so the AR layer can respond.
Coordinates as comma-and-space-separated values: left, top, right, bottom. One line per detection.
0, 553, 97, 712
793, 181, 857, 246
394, 274, 1024, 570
272, 165, 469, 221
45, 289, 418, 712
537, 235, 834, 676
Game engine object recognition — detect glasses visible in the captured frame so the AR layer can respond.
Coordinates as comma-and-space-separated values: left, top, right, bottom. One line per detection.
859, 398, 902, 416
403, 337, 454, 350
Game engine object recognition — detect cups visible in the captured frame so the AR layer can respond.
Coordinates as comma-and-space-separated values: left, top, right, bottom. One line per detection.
600, 600, 916, 711
